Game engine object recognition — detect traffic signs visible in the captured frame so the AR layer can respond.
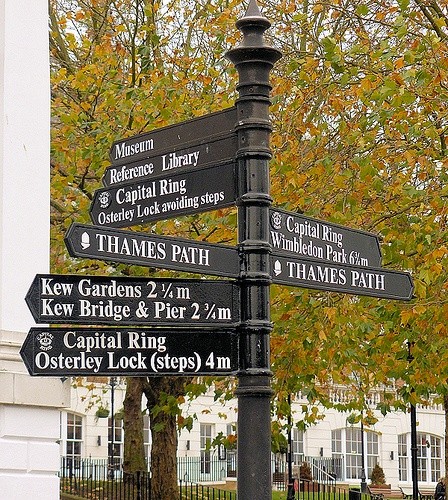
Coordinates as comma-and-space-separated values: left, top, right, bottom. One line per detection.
267, 206, 383, 269
25, 274, 240, 326
63, 222, 240, 279
87, 158, 236, 228
269, 254, 414, 300
109, 107, 238, 166
19, 327, 239, 375
100, 134, 237, 188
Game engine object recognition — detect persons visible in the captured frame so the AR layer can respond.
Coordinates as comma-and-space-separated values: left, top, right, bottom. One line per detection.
434, 477, 446, 500
